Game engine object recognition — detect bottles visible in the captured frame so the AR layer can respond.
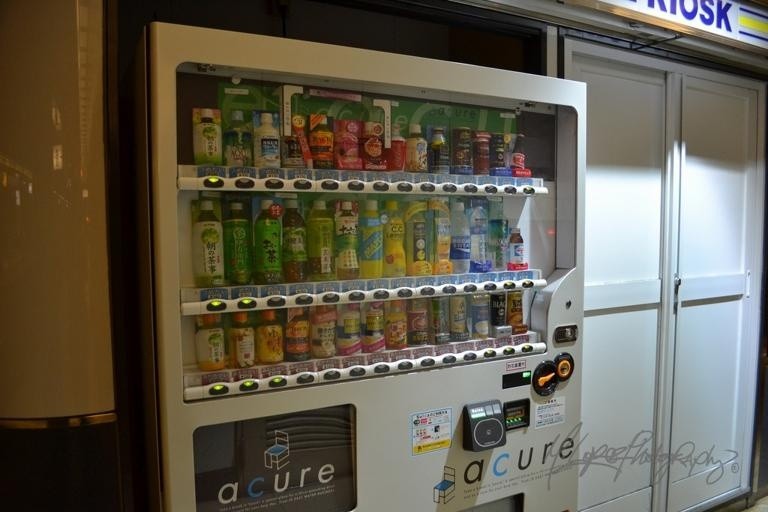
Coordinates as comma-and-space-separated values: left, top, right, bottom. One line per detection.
191, 110, 528, 371
